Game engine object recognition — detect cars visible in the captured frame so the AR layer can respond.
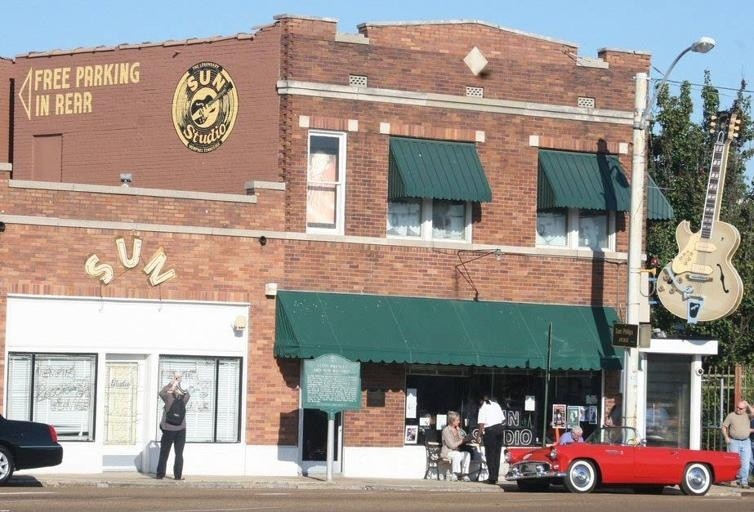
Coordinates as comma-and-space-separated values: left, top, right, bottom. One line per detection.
0, 414, 63, 487
503, 426, 742, 496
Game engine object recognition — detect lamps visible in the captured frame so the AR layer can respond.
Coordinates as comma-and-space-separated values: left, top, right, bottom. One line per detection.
457, 249, 501, 253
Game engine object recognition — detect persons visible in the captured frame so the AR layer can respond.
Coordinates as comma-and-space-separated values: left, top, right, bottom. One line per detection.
557, 425, 584, 444
646, 395, 672, 435
476, 393, 505, 486
438, 410, 472, 483
747, 405, 753, 476
605, 392, 621, 444
155, 375, 191, 482
720, 401, 754, 490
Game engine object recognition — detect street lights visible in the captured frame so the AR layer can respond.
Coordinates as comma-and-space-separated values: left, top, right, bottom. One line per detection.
624, 37, 715, 446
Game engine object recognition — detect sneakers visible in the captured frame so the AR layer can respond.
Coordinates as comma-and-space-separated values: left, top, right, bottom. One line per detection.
728, 482, 750, 488
451, 476, 498, 485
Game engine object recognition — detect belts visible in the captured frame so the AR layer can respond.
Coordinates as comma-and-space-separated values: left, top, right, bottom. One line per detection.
729, 435, 749, 441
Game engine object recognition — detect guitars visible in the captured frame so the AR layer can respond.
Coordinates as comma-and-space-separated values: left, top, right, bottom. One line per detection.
656, 111, 744, 322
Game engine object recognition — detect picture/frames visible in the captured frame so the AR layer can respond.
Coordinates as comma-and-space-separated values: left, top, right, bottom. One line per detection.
552, 404, 597, 429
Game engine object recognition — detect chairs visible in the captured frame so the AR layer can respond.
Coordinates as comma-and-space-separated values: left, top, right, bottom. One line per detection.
424, 431, 448, 481
455, 428, 487, 482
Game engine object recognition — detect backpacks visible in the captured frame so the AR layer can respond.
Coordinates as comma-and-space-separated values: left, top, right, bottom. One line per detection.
166, 393, 185, 426
